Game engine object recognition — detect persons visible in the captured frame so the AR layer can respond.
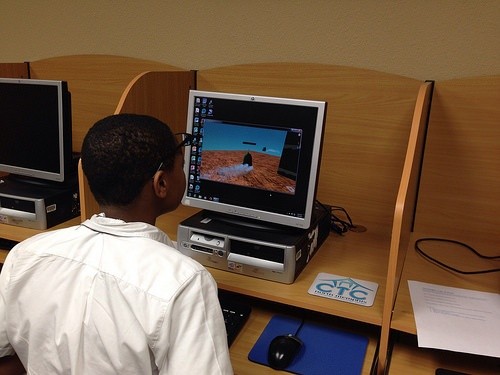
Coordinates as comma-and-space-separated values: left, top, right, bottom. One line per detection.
0, 113, 234, 375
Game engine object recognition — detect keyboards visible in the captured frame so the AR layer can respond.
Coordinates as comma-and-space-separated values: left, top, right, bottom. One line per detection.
217, 295, 254, 348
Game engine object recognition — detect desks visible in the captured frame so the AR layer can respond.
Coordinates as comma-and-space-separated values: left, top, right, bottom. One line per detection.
0, 184, 500, 375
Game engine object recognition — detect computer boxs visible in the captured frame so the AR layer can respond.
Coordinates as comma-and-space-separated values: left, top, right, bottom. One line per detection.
177, 203, 332, 284
0, 150, 81, 230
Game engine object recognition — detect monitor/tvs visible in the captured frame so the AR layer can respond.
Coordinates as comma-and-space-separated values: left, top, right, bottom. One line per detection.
0, 78, 71, 182
179, 89, 327, 229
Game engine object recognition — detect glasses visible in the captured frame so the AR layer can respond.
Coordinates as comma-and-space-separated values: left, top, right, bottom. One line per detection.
152, 132, 193, 179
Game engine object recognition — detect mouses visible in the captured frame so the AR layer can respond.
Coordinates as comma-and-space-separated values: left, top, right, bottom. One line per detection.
267, 333, 302, 370
